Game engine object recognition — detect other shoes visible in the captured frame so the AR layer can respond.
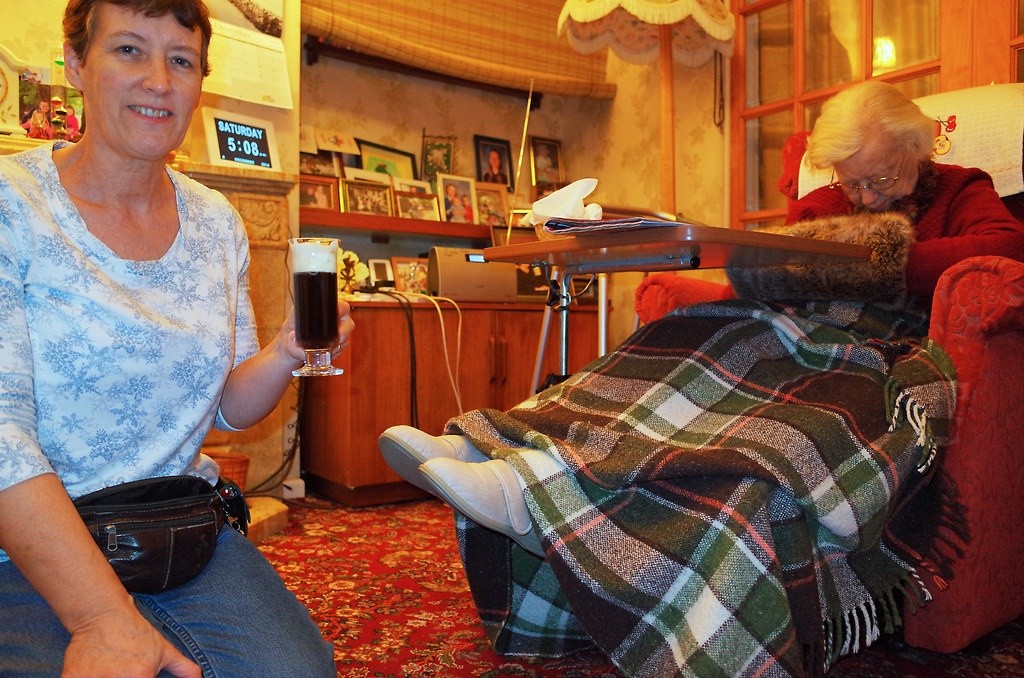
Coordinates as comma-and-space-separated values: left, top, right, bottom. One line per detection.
417, 458, 547, 560
377, 425, 489, 496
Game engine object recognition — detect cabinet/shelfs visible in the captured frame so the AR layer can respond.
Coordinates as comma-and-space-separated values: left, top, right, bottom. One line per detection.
301, 307, 611, 507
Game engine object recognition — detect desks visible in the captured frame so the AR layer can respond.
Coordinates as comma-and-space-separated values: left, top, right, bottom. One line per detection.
483, 225, 869, 395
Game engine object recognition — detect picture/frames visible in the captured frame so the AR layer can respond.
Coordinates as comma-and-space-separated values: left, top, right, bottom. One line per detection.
422, 135, 461, 181
299, 174, 340, 211
342, 166, 391, 184
391, 176, 433, 194
472, 134, 516, 192
355, 137, 418, 180
475, 180, 515, 226
527, 135, 567, 186
391, 256, 429, 293
394, 190, 443, 222
299, 149, 346, 178
435, 172, 479, 223
490, 226, 577, 305
339, 178, 397, 217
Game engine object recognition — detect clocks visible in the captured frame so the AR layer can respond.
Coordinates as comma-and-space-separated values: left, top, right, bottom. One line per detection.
0, 44, 29, 137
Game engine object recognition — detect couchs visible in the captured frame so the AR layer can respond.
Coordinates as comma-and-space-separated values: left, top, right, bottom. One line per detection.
632, 80, 1024, 655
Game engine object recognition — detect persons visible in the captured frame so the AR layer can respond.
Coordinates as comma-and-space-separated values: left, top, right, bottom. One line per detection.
378, 79, 1024, 559
350, 189, 384, 212
301, 156, 321, 175
376, 164, 388, 174
406, 186, 425, 212
21, 100, 78, 142
445, 184, 473, 223
428, 147, 448, 173
0, 1, 355, 678
537, 146, 558, 182
484, 152, 507, 185
301, 185, 328, 207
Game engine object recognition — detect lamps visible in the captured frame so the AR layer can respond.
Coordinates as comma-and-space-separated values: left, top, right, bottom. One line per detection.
556, 0, 736, 215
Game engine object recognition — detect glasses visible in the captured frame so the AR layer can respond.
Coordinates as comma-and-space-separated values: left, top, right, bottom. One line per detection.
829, 156, 905, 193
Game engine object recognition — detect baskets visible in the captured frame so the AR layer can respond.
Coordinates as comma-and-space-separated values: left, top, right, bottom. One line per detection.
206, 451, 250, 489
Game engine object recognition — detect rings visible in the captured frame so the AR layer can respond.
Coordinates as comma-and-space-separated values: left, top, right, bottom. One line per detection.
338, 344, 341, 353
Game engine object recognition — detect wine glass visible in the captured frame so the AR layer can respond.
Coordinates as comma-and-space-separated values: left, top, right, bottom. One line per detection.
287, 237, 344, 376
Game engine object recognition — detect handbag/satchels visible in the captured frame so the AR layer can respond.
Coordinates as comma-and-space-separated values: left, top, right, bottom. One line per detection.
73, 475, 227, 594
